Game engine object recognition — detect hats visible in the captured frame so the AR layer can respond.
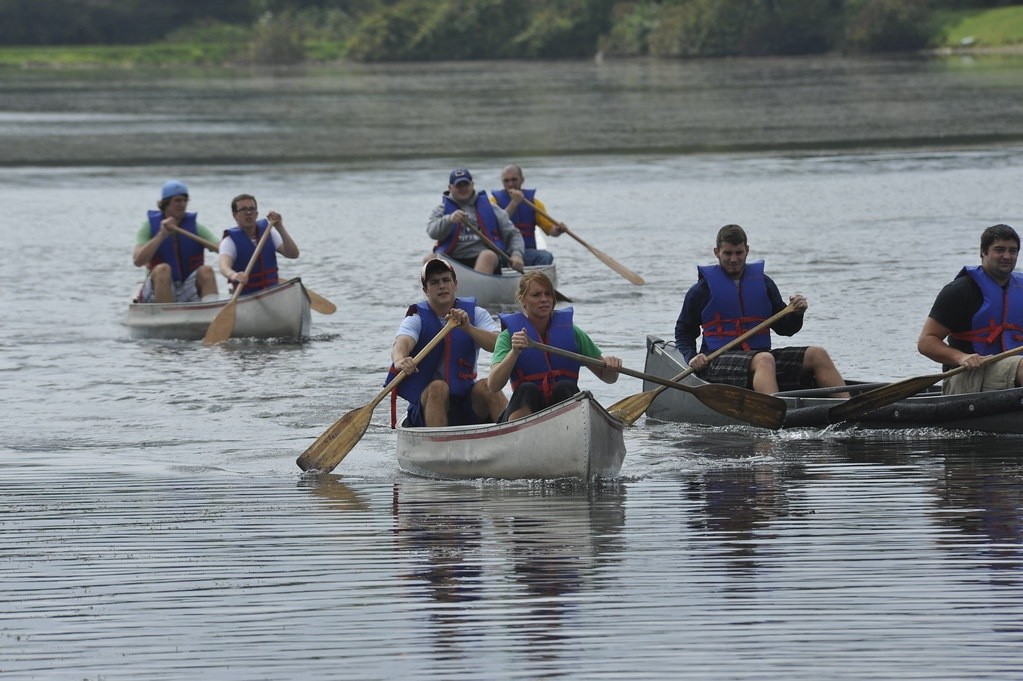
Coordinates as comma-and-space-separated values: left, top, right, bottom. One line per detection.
161, 180, 189, 201
421, 258, 456, 282
449, 168, 472, 187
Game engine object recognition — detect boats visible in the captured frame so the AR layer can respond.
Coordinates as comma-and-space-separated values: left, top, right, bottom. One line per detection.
395, 366, 628, 485
420, 249, 557, 315
643, 335, 1023, 438
125, 270, 312, 343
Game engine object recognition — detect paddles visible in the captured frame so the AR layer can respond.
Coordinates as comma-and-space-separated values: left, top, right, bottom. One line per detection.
166, 219, 340, 315
508, 186, 646, 284
602, 302, 798, 421
524, 335, 786, 430
295, 320, 459, 474
459, 217, 572, 301
203, 215, 277, 346
829, 341, 1023, 419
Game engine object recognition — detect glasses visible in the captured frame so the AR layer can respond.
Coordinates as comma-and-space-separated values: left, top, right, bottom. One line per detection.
236, 207, 256, 212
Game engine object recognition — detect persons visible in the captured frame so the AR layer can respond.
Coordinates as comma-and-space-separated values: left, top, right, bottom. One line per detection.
674, 224, 850, 398
218, 194, 300, 296
426, 169, 524, 274
133, 181, 220, 303
391, 259, 509, 427
488, 270, 622, 422
489, 164, 567, 266
918, 224, 1023, 394
